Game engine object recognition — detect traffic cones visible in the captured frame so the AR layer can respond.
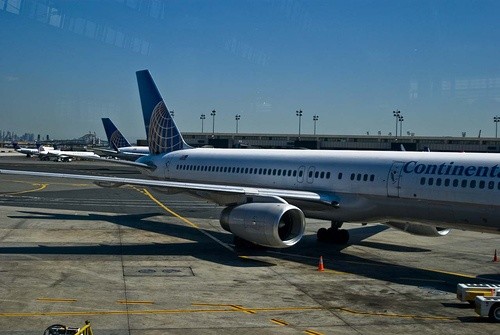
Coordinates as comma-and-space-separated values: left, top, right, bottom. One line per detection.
316, 255, 326, 271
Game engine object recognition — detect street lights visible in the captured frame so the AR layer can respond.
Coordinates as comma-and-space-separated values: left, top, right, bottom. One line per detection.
296, 110, 303, 138
211, 110, 216, 136
313, 115, 319, 137
399, 115, 404, 136
235, 114, 241, 133
200, 115, 205, 134
494, 116, 500, 139
393, 110, 401, 138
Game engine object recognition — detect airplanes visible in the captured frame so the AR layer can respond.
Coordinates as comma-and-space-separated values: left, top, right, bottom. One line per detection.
0, 68, 500, 253
16, 146, 105, 162
